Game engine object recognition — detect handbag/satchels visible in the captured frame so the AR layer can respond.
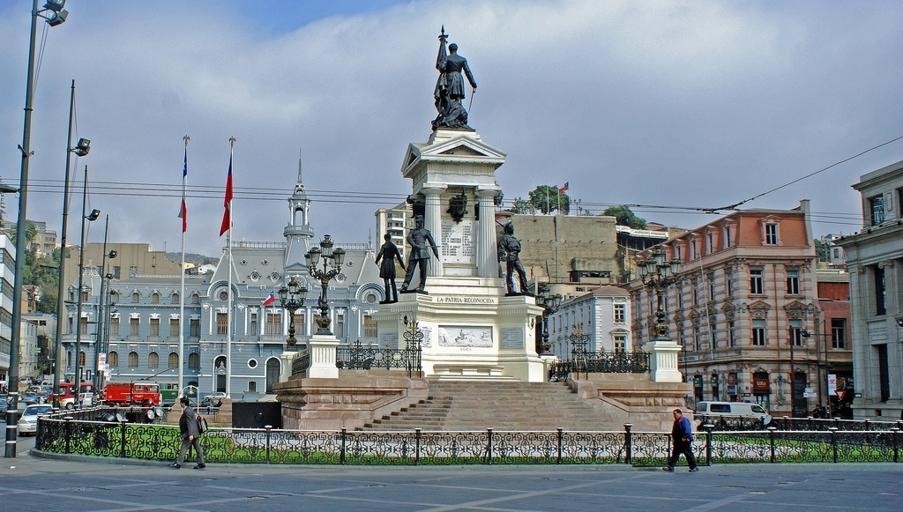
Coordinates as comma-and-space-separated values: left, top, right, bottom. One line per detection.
196, 414, 208, 433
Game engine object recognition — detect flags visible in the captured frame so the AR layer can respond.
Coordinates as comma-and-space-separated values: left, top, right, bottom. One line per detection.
219, 144, 233, 237
558, 181, 569, 193
261, 290, 274, 306
177, 143, 187, 232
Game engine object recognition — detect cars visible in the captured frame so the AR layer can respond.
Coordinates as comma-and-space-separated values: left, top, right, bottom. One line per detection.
203, 391, 228, 405
0, 375, 101, 438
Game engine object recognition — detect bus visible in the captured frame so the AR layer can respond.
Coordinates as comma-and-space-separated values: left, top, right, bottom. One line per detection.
137, 381, 181, 400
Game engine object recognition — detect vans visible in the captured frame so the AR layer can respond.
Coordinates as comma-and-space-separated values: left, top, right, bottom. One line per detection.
697, 400, 772, 429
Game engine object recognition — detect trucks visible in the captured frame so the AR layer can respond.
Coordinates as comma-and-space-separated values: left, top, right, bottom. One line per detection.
102, 381, 160, 409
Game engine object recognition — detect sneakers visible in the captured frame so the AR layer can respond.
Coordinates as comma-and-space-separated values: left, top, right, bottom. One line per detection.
662, 466, 675, 473
172, 462, 180, 470
688, 467, 700, 472
192, 463, 206, 469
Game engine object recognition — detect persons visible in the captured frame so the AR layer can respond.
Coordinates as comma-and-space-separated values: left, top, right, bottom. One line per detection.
662, 409, 699, 472
374, 233, 406, 304
431, 43, 477, 130
497, 221, 532, 295
397, 215, 439, 292
168, 397, 207, 470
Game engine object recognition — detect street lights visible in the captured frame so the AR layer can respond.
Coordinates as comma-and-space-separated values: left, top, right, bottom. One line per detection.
94, 210, 118, 398
302, 235, 345, 338
798, 318, 835, 419
75, 163, 99, 406
53, 74, 89, 414
535, 293, 563, 355
278, 276, 307, 351
634, 249, 681, 344
0, 0, 68, 460
